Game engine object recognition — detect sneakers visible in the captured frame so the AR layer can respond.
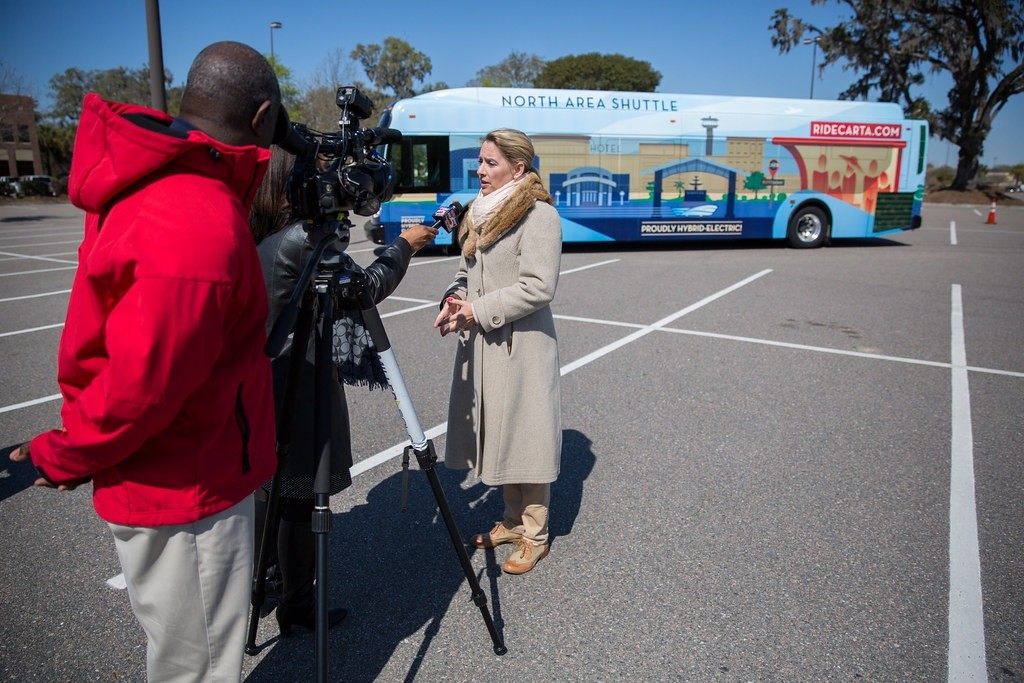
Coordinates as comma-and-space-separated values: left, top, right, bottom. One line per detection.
469, 522, 524, 549
503, 539, 549, 574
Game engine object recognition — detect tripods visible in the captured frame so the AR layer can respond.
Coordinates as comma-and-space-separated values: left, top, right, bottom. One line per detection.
246, 216, 506, 683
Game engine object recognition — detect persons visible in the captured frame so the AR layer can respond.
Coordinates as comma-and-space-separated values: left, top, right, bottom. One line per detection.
433, 128, 562, 576
247, 127, 439, 597
10, 38, 283, 683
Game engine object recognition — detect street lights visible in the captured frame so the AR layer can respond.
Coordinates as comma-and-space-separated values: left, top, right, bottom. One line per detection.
269, 21, 284, 72
802, 36, 824, 99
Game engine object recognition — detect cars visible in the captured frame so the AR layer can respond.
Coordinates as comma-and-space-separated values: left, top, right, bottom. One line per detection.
0, 170, 71, 199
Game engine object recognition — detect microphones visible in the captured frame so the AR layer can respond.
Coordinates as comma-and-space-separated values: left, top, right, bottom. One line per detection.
431, 201, 465, 233
360, 128, 402, 145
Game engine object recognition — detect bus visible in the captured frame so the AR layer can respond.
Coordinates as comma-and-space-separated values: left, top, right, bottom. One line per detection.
363, 85, 931, 258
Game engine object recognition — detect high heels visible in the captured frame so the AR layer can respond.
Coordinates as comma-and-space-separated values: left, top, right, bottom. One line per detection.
276, 596, 347, 638
250, 571, 284, 605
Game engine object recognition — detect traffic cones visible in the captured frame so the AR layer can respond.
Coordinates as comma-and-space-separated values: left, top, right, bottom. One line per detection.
983, 196, 998, 225
